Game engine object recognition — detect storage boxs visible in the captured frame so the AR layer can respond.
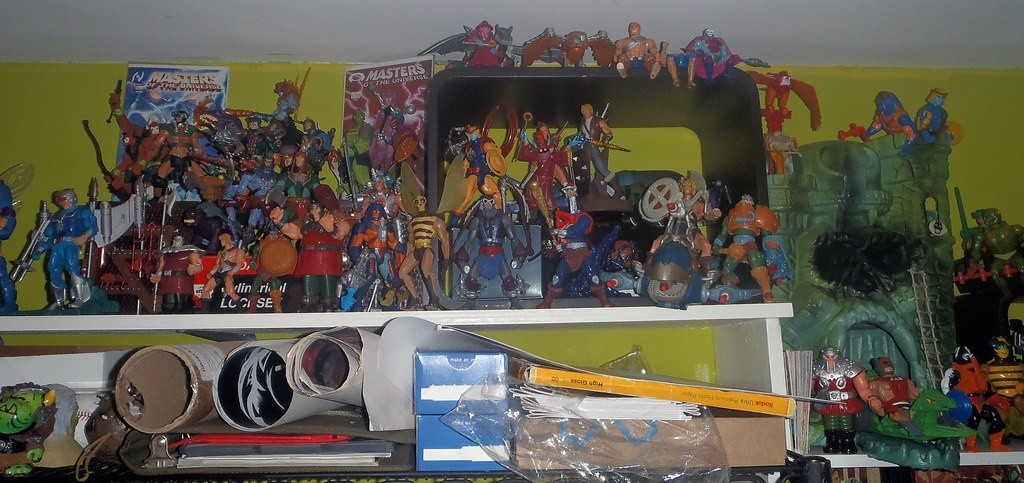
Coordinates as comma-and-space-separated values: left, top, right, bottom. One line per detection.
510, 360, 798, 420
415, 414, 512, 472
513, 406, 788, 471
413, 347, 509, 416
0, 344, 151, 451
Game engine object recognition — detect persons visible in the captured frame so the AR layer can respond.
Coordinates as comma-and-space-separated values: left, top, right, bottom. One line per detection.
862, 92, 919, 157
453, 104, 626, 308
105, 92, 451, 312
986, 336, 1024, 422
32, 189, 98, 310
614, 22, 735, 90
869, 357, 919, 422
766, 131, 798, 172
0, 180, 19, 311
645, 179, 776, 303
916, 88, 948, 143
963, 207, 1024, 302
467, 21, 505, 66
811, 348, 882, 454
941, 346, 1014, 452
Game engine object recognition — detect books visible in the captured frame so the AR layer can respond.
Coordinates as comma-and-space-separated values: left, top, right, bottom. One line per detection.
509, 388, 702, 419
